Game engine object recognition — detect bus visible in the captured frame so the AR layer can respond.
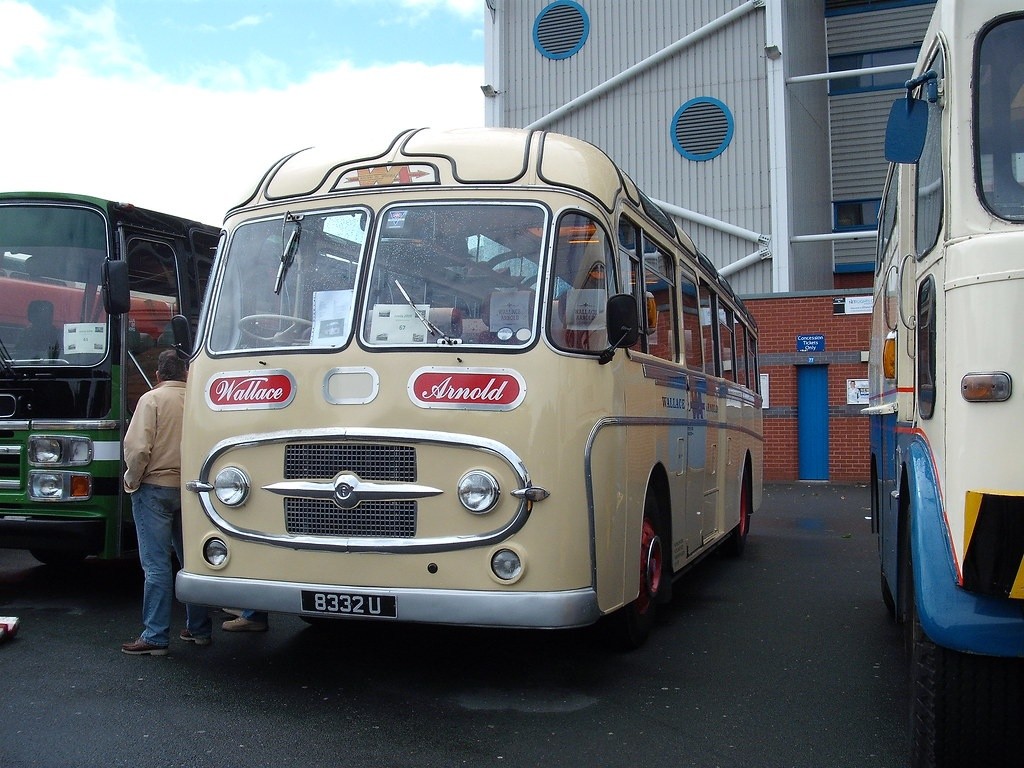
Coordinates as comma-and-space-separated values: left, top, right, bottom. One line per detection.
174, 128, 767, 654
1, 193, 475, 564
868, 0, 1022, 662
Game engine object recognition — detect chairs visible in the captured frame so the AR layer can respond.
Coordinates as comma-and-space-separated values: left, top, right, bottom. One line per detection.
557, 289, 608, 350
478, 290, 536, 345
127, 321, 173, 353
461, 318, 489, 344
429, 308, 463, 343
550, 300, 563, 342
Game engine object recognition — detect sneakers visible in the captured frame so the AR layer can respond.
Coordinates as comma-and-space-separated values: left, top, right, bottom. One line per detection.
180, 629, 212, 644
223, 616, 267, 630
223, 608, 243, 616
122, 637, 167, 655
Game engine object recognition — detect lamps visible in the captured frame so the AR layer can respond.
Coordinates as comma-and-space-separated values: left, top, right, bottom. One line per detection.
480, 84, 498, 97
764, 44, 781, 60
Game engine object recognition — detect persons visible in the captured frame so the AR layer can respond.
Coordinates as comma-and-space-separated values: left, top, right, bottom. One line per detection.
847, 381, 860, 404
122, 349, 212, 655
222, 608, 269, 631
